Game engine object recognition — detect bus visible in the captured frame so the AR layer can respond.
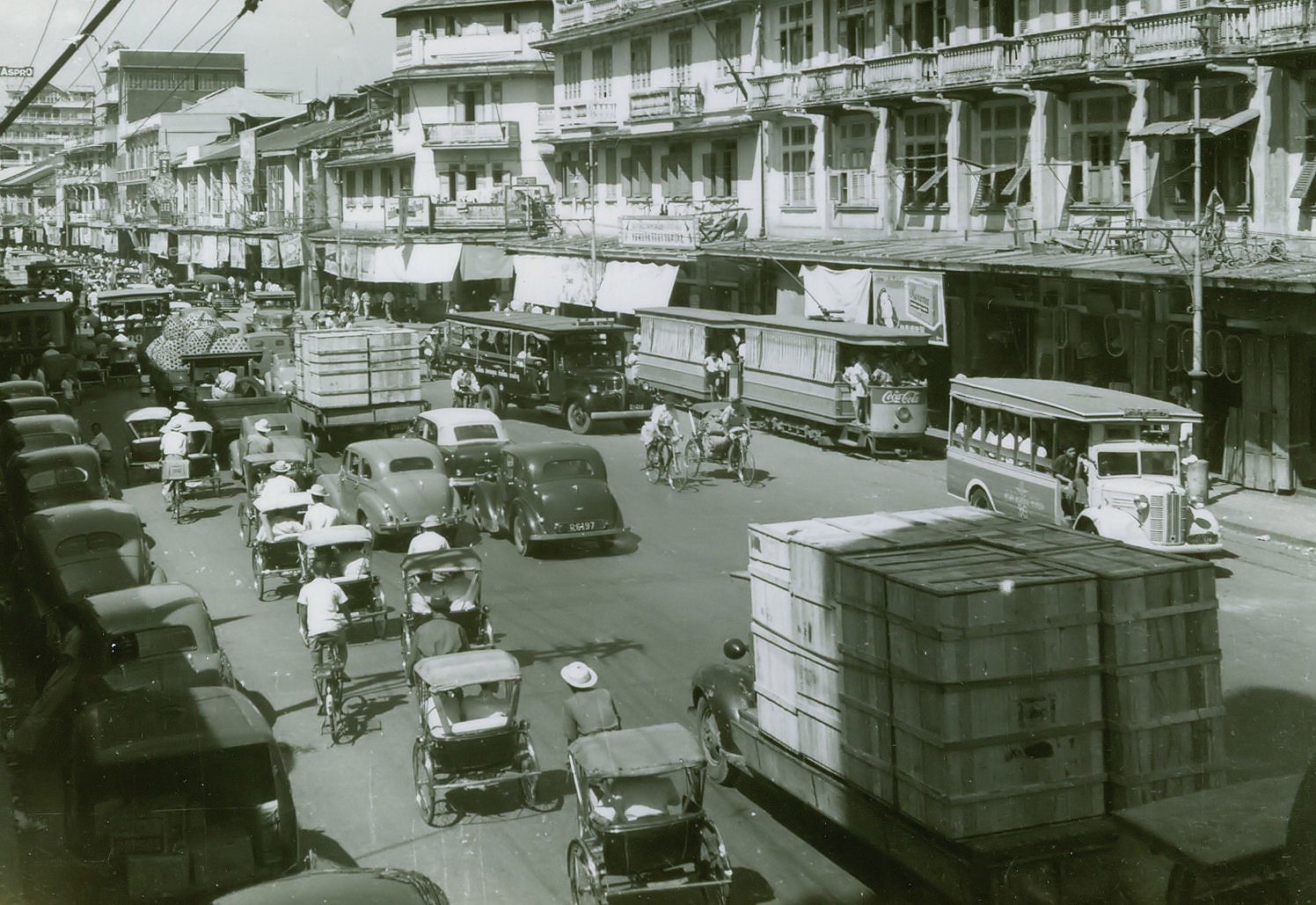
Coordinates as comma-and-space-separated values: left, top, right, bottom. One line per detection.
945, 372, 1226, 557
634, 306, 930, 460
442, 310, 656, 436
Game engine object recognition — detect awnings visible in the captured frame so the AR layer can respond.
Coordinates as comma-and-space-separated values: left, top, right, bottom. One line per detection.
1128, 109, 1261, 140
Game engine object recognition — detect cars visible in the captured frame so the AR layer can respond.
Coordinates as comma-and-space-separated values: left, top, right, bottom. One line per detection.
55, 583, 236, 758
8, 439, 122, 529
403, 405, 513, 501
468, 437, 630, 558
1106, 758, 1315, 905
1, 247, 297, 470
310, 438, 469, 554
59, 679, 304, 905
207, 860, 449, 905
7, 497, 174, 663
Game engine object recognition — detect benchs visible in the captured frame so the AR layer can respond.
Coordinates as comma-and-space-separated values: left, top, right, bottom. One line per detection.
587, 795, 705, 873
188, 453, 211, 476
80, 368, 103, 380
131, 437, 162, 462
259, 540, 306, 569
333, 572, 374, 610
427, 721, 519, 772
261, 471, 318, 491
409, 602, 480, 643
110, 360, 135, 376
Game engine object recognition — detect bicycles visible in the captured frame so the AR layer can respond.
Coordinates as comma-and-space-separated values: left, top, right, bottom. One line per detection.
303, 624, 351, 745
639, 429, 693, 492
155, 452, 191, 526
451, 387, 479, 411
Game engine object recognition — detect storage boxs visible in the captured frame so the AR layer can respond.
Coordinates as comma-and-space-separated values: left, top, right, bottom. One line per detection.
125, 829, 255, 895
749, 505, 1232, 840
294, 327, 421, 408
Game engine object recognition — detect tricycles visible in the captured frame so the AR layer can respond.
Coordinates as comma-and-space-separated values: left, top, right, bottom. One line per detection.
118, 405, 224, 497
685, 401, 757, 488
396, 545, 500, 684
563, 719, 735, 905
400, 631, 542, 824
232, 447, 393, 642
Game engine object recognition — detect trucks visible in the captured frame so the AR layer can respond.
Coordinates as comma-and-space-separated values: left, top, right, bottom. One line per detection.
267, 354, 435, 456
687, 568, 1120, 905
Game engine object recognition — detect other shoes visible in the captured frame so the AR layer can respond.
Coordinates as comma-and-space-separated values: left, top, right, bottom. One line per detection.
317, 702, 328, 715
335, 672, 352, 682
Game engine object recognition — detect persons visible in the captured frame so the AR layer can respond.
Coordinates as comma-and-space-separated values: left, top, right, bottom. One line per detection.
244, 420, 372, 577
627, 326, 640, 383
955, 412, 1087, 508
12, 303, 236, 510
876, 289, 901, 327
704, 342, 745, 399
5, 242, 282, 310
319, 279, 548, 408
561, 661, 622, 744
651, 394, 682, 468
409, 516, 479, 614
840, 352, 903, 425
297, 560, 355, 713
722, 394, 752, 472
406, 596, 507, 735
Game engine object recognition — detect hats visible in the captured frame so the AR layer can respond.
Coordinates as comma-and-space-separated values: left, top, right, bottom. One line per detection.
459, 360, 470, 367
421, 514, 444, 528
560, 661, 597, 688
174, 401, 190, 410
118, 328, 125, 332
46, 341, 56, 347
328, 310, 335, 315
730, 394, 742, 403
307, 484, 330, 496
665, 393, 676, 405
167, 419, 184, 430
220, 359, 231, 367
255, 419, 271, 432
270, 461, 291, 472
429, 328, 440, 335
428, 594, 454, 610
